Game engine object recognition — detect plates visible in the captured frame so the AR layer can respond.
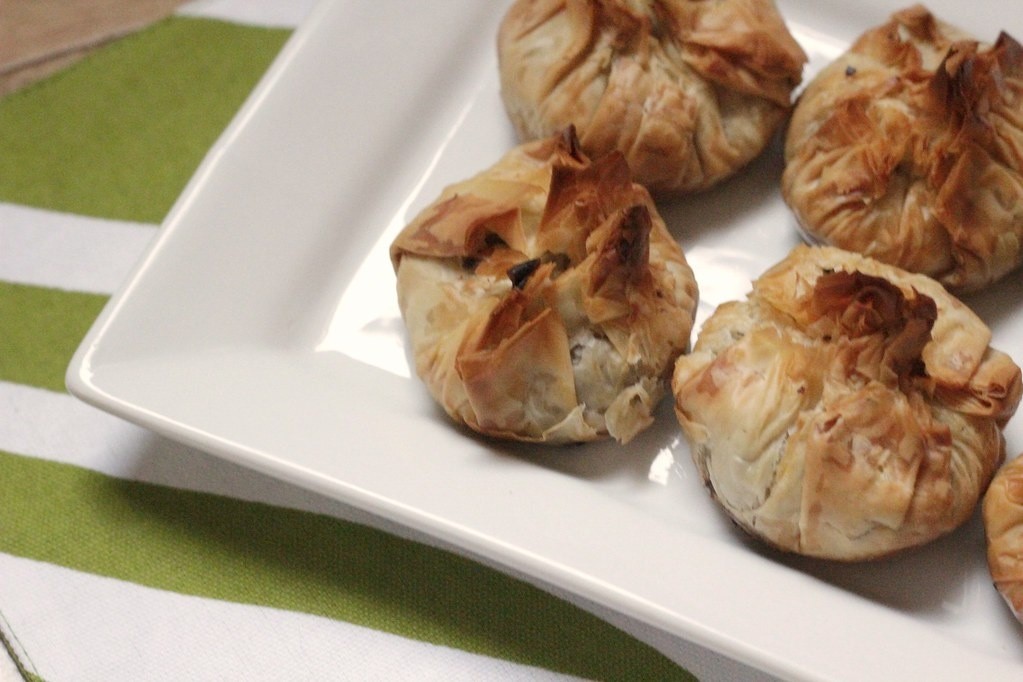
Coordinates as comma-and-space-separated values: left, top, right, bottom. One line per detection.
66, 0, 1023, 682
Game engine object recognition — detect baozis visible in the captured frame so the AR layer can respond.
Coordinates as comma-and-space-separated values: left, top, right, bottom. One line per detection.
387, 0, 1023, 630
